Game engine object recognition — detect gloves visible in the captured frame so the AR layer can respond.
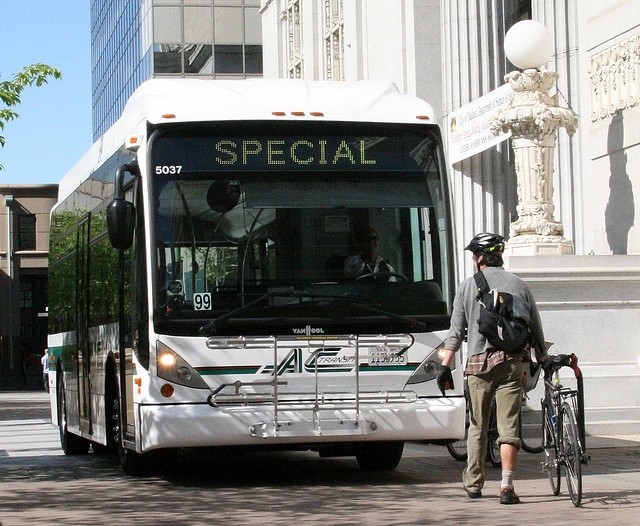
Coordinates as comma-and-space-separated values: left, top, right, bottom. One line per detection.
540, 353, 570, 370
437, 365, 454, 397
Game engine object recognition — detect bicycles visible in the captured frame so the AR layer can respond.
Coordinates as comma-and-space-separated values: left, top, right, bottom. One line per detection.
538, 358, 591, 507
447, 391, 523, 467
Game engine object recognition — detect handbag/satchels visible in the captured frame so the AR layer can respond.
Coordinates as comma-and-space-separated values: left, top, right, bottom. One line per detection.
473, 271, 531, 354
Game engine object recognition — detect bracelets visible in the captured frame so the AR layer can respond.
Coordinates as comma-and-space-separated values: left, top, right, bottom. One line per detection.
539, 355, 550, 366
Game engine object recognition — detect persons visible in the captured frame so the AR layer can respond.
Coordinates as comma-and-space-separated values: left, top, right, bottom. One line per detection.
41, 349, 49, 393
343, 226, 397, 282
437, 233, 561, 504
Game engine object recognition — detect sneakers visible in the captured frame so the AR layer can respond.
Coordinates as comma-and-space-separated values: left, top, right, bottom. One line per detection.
500, 486, 520, 504
463, 485, 481, 498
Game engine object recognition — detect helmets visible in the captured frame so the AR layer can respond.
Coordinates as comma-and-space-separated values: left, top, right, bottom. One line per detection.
464, 233, 504, 256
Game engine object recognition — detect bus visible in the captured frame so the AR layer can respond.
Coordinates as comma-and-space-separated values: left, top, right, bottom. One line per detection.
46, 79, 466, 475
